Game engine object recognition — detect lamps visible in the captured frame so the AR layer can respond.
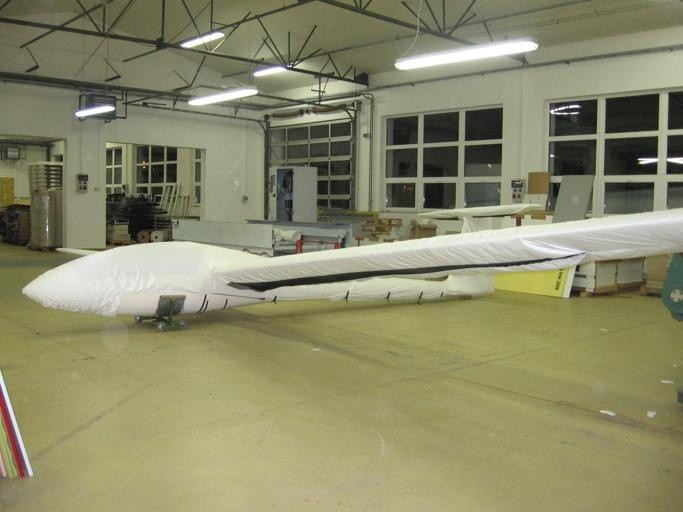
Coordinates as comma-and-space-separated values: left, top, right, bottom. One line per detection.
392, 1, 540, 72
185, 17, 262, 108
